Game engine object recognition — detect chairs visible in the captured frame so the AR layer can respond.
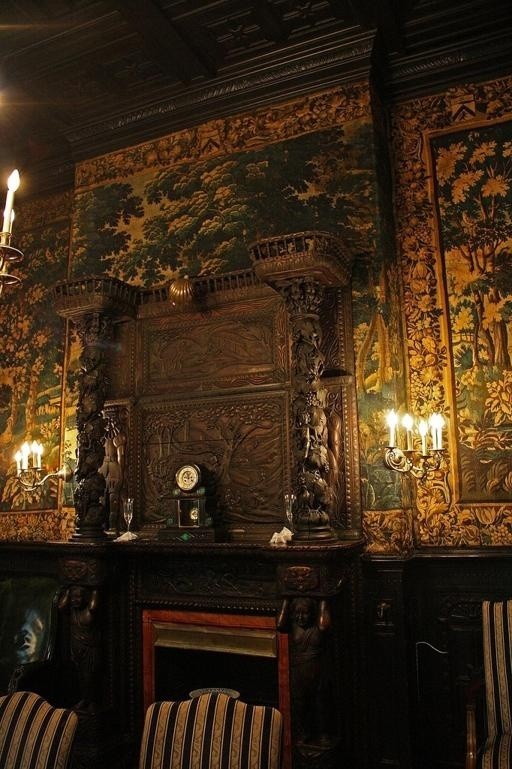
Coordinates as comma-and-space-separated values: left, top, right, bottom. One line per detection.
0, 688, 84, 769
129, 689, 285, 768
475, 594, 512, 768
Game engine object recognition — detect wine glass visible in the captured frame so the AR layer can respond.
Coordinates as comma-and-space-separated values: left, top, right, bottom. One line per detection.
119, 496, 135, 543
283, 494, 298, 535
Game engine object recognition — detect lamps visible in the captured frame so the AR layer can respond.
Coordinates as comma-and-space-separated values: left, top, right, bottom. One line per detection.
379, 405, 451, 484
1, 166, 28, 290
11, 436, 72, 498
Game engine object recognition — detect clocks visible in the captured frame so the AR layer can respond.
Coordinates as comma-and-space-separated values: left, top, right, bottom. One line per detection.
157, 462, 220, 541
